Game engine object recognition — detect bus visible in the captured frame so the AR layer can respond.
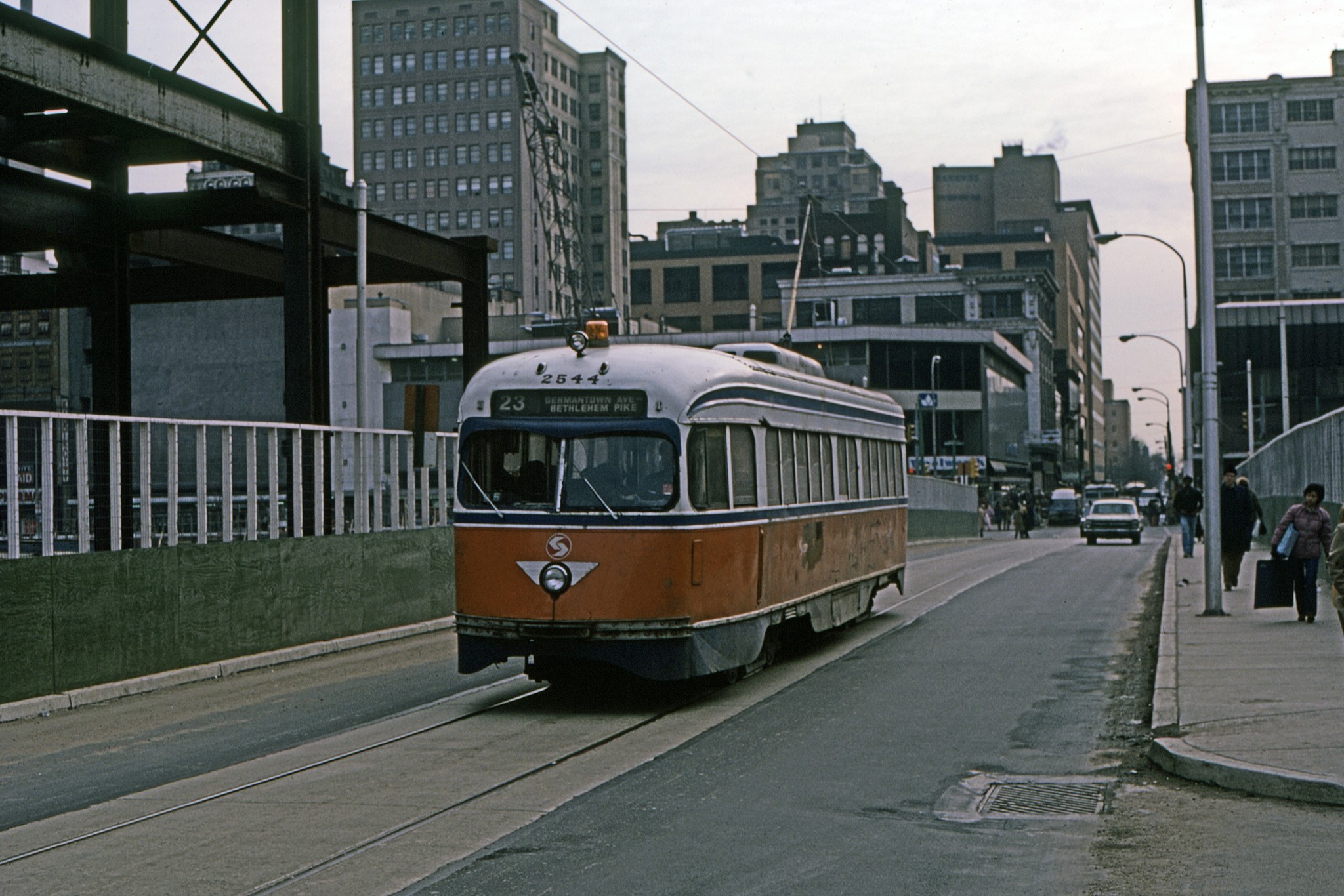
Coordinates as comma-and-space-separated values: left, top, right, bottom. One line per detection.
452, 190, 911, 688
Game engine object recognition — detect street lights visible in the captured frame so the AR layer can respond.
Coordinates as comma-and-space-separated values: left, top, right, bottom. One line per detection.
1118, 332, 1188, 475
1092, 230, 1193, 478
1131, 386, 1176, 480
931, 355, 942, 477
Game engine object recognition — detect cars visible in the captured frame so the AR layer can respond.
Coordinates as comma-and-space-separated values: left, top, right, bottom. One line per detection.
1047, 480, 1171, 527
1080, 498, 1145, 546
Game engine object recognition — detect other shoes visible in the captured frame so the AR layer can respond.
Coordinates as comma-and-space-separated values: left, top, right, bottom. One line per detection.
1224, 587, 1233, 592
1306, 617, 1315, 624
1298, 614, 1306, 623
1183, 552, 1193, 558
1231, 578, 1238, 586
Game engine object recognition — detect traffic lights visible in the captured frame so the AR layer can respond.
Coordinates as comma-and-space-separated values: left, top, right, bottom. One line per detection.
1241, 411, 1249, 431
1166, 462, 1174, 480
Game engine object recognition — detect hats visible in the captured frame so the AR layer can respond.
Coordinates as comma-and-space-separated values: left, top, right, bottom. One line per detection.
1304, 484, 1324, 502
1223, 463, 1238, 476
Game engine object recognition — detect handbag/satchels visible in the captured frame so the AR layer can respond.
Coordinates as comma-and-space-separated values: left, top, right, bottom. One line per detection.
1277, 523, 1298, 556
1254, 560, 1295, 608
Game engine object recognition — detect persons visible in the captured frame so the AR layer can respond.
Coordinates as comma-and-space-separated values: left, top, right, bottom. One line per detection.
1173, 466, 1344, 634
979, 486, 1051, 539
